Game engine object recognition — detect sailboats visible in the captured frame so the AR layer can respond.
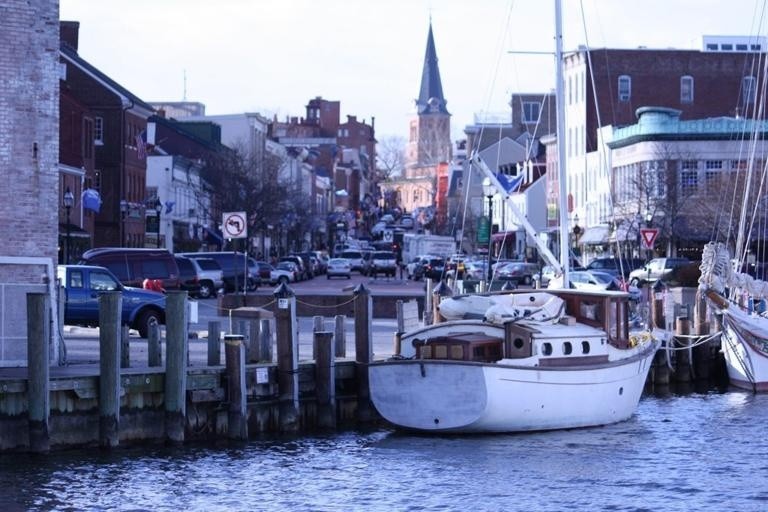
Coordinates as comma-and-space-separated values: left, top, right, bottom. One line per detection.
369, 1, 674, 433
694, 46, 768, 393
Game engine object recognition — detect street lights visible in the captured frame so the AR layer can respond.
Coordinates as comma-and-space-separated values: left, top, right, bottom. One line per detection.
481, 176, 500, 271
152, 196, 163, 248
62, 186, 75, 265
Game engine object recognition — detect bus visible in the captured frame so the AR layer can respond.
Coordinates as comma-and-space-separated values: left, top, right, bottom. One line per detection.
400, 233, 456, 268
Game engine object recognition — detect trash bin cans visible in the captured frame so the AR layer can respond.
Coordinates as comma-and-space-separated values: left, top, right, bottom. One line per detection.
231, 306, 272, 363
675, 316, 689, 335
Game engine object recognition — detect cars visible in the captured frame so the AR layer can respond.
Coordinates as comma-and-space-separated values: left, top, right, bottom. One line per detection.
405, 252, 646, 304
371, 205, 413, 237
257, 239, 398, 287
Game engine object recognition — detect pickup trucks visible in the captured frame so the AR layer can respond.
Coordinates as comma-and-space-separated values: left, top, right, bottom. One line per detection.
627, 257, 690, 288
51, 265, 167, 338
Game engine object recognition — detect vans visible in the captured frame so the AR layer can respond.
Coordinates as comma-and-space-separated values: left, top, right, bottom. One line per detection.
77, 247, 261, 299
